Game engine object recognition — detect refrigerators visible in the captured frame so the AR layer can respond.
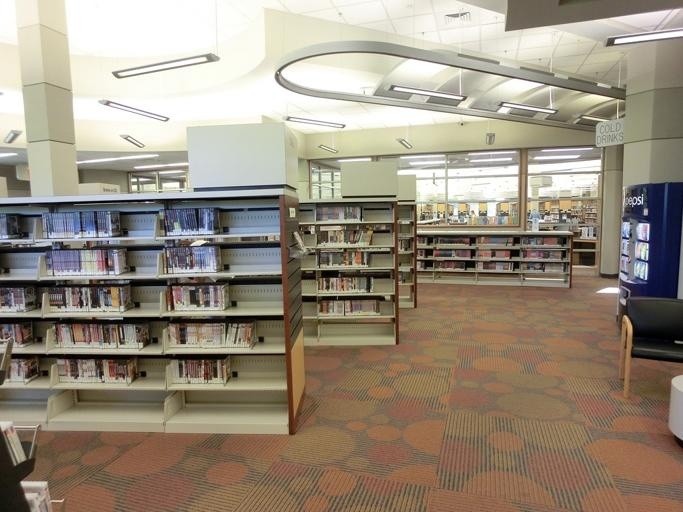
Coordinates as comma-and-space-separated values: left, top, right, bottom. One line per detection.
619, 185, 678, 313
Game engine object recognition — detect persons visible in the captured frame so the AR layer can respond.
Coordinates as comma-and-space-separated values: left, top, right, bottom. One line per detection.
418, 207, 570, 225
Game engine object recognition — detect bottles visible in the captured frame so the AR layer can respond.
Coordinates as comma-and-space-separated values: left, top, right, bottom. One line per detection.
634, 220, 648, 279
620, 223, 633, 271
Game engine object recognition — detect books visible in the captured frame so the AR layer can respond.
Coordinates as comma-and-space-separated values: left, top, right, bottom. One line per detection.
45, 241, 128, 275
170, 354, 232, 384
41, 210, 121, 239
54, 321, 151, 348
1, 286, 37, 312
0, 420, 56, 512
1, 320, 33, 344
167, 278, 230, 311
57, 355, 139, 384
571, 200, 598, 234
314, 205, 381, 316
292, 231, 307, 252
48, 279, 133, 312
162, 239, 224, 274
2, 357, 40, 380
398, 234, 567, 283
159, 209, 223, 236
1, 214, 22, 237
167, 317, 257, 348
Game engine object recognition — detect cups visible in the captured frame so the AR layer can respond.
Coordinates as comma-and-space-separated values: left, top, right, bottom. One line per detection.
588, 227, 592, 237
582, 229, 587, 237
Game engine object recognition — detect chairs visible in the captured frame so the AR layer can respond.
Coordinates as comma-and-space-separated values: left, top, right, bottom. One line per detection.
621, 297, 682, 400
